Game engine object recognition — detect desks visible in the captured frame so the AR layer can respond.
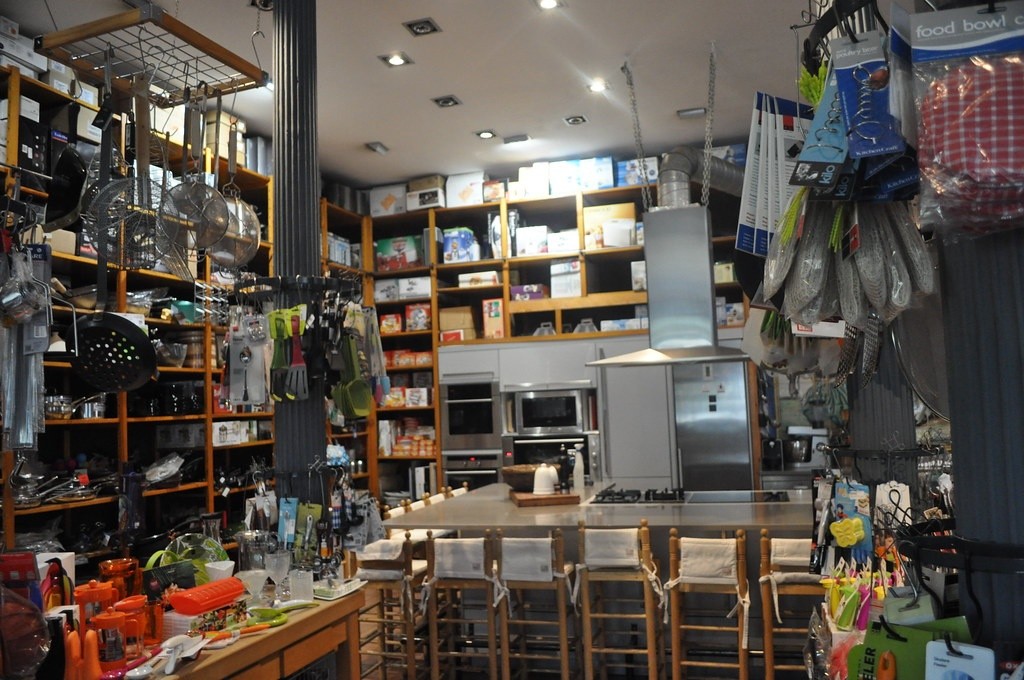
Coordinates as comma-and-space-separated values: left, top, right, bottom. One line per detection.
126, 564, 363, 680
382, 495, 814, 680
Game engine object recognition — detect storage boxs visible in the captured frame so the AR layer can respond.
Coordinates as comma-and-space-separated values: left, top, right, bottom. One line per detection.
0, 15, 276, 175
21, 220, 77, 254
1, 94, 74, 180
434, 204, 651, 343
155, 420, 258, 449
370, 158, 659, 213
373, 224, 440, 456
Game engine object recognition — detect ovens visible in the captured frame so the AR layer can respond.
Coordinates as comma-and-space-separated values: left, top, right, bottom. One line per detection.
439, 375, 592, 502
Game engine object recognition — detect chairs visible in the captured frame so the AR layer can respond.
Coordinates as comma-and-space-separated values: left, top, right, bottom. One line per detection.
359, 483, 827, 680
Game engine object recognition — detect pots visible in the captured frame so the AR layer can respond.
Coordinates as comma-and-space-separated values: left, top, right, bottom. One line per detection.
44, 393, 106, 419
49, 276, 96, 310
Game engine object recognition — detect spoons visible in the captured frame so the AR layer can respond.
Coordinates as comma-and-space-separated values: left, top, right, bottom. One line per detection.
102, 647, 164, 680
126, 648, 170, 679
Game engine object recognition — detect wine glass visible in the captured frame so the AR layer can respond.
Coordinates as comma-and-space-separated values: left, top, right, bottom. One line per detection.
234, 569, 272, 606
264, 548, 291, 603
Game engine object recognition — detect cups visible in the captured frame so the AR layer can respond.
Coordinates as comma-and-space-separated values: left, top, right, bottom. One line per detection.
289, 564, 313, 604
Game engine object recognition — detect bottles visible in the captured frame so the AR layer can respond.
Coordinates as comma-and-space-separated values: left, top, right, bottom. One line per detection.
572, 443, 586, 489
559, 443, 570, 489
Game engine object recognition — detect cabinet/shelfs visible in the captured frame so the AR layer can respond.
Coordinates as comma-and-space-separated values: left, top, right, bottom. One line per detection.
317, 201, 379, 506
0, 66, 275, 576
370, 184, 758, 493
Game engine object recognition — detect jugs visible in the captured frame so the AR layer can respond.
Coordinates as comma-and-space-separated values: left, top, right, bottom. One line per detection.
73, 557, 161, 674
232, 529, 280, 586
